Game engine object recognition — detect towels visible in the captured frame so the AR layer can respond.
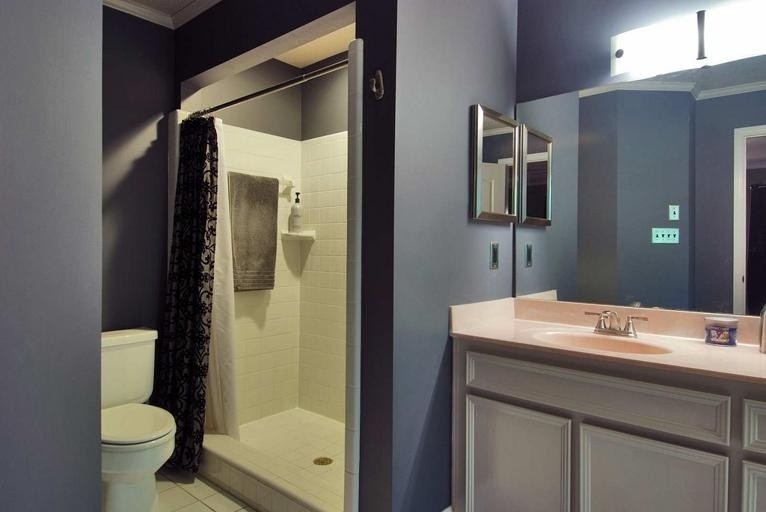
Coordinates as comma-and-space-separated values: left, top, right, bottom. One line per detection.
229, 170, 280, 292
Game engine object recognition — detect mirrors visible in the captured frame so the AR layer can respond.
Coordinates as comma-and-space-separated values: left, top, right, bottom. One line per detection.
520, 123, 554, 228
466, 101, 520, 224
514, 54, 765, 317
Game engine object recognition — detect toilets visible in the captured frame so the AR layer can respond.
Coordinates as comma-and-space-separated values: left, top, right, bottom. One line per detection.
102, 326, 177, 511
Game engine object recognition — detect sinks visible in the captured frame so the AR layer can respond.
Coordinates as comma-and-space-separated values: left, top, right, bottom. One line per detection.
535, 328, 674, 356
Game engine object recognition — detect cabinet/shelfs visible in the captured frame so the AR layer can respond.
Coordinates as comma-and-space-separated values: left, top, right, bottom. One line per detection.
576, 422, 732, 512
462, 391, 576, 511
733, 457, 766, 511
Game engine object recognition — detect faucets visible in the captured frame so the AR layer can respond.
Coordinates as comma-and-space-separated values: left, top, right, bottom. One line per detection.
601, 310, 621, 331
625, 300, 643, 308
